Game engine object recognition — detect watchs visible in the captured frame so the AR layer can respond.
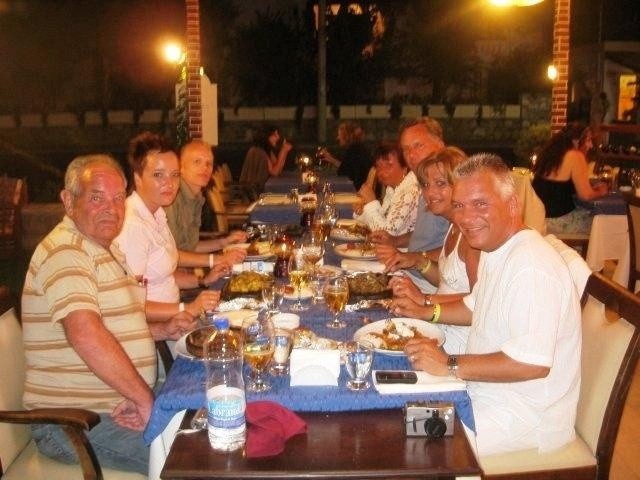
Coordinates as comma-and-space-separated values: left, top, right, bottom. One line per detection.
424, 294, 431, 307
446, 355, 458, 378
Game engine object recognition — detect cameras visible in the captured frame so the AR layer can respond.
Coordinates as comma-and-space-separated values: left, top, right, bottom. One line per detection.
404, 400, 454, 438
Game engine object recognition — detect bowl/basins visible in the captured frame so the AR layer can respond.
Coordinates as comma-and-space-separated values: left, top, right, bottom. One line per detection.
271, 313, 300, 331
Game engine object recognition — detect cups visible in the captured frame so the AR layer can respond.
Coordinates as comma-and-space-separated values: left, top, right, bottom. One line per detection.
267, 335, 290, 377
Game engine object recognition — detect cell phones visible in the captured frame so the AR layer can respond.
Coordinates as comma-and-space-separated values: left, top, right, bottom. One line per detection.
376, 371, 417, 384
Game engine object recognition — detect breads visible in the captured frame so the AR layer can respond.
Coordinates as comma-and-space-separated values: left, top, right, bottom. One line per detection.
185, 326, 253, 359
368, 326, 424, 350
247, 239, 273, 255
346, 272, 395, 304
220, 270, 270, 299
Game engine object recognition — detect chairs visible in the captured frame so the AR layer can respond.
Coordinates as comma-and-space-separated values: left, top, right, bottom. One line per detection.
453, 272, 640, 480
509, 172, 589, 260
0, 173, 28, 268
0, 287, 146, 480
622, 192, 640, 292
199, 162, 259, 240
586, 215, 631, 289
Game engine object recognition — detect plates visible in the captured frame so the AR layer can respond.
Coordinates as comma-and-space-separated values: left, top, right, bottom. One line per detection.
174, 330, 246, 361
341, 258, 404, 277
224, 262, 276, 281
330, 228, 366, 241
336, 218, 363, 225
223, 243, 274, 262
334, 241, 377, 258
352, 318, 447, 359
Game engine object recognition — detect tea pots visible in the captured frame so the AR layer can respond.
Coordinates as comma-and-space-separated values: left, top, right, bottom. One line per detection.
270, 234, 297, 260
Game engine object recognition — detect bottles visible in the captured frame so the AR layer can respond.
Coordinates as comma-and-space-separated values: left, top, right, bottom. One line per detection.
203, 316, 247, 454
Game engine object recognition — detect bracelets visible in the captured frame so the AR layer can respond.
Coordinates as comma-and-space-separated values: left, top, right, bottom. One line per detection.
198, 276, 209, 288
421, 251, 426, 255
430, 303, 441, 323
416, 257, 431, 273
209, 254, 213, 269
178, 302, 184, 312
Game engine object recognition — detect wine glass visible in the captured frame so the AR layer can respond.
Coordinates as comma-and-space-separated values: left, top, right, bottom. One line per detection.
259, 281, 286, 313
314, 143, 329, 170
283, 182, 350, 330
240, 314, 278, 393
344, 339, 375, 391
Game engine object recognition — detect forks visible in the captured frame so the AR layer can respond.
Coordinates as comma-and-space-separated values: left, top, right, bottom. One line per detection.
385, 319, 408, 341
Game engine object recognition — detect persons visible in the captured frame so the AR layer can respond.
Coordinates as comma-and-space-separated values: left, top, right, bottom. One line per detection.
21, 153, 167, 476
112, 133, 247, 376
370, 148, 482, 358
367, 116, 449, 295
349, 141, 421, 237
162, 141, 246, 291
531, 122, 609, 234
321, 121, 382, 200
237, 123, 291, 204
405, 156, 582, 458
